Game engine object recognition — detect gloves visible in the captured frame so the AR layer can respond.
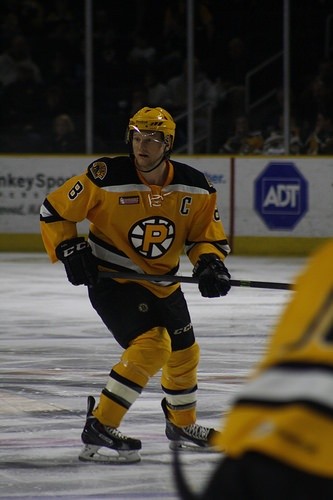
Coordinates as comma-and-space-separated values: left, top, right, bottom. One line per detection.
55, 236, 99, 286
192, 253, 231, 298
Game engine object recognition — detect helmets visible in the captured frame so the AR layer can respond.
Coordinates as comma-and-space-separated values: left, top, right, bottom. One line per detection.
127, 107, 176, 149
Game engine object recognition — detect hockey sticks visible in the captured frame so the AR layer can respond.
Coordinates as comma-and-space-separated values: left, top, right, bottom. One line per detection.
97, 268, 296, 292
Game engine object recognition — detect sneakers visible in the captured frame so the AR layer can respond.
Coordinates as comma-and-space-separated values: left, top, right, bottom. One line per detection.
78, 396, 142, 465
161, 397, 225, 453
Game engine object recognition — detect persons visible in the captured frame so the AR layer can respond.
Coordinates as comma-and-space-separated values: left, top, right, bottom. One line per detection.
38, 107, 232, 464
50, 115, 74, 153
203, 239, 333, 500
218, 117, 333, 155
